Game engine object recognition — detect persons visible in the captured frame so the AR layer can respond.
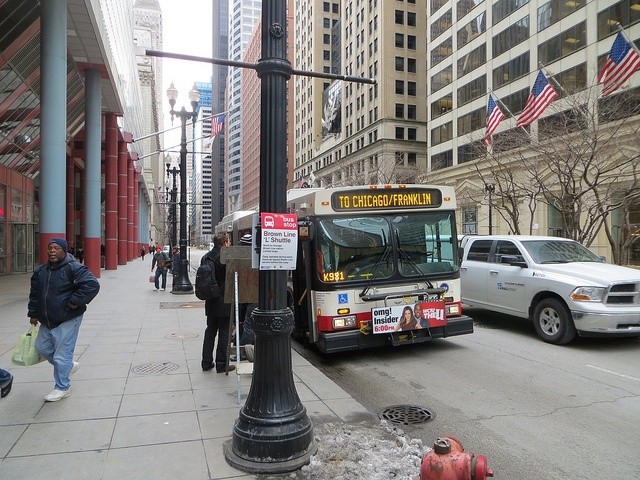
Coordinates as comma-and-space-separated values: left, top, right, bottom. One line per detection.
170, 247, 180, 293
152, 245, 155, 255
151, 246, 170, 291
201, 231, 236, 373
68, 247, 74, 255
78, 246, 83, 264
140, 246, 145, 261
149, 245, 151, 254
395, 308, 419, 332
26, 238, 100, 402
413, 305, 430, 330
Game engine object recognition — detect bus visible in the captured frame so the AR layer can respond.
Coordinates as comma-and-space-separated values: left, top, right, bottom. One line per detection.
215, 185, 474, 354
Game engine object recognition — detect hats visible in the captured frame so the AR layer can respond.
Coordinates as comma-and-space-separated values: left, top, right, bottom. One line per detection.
240, 234, 252, 244
48, 238, 66, 253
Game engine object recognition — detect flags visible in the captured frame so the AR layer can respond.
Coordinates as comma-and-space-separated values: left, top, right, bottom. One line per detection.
599, 31, 640, 98
209, 114, 225, 140
483, 93, 506, 147
515, 67, 559, 129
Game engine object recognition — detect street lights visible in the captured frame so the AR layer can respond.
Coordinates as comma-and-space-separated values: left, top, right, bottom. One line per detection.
168, 83, 199, 294
163, 152, 181, 269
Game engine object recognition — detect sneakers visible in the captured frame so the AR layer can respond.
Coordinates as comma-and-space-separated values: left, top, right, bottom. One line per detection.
71, 362, 80, 375
160, 288, 165, 290
44, 388, 70, 402
154, 287, 157, 290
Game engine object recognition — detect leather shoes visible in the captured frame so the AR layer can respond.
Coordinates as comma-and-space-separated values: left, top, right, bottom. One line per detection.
204, 362, 214, 369
220, 365, 235, 372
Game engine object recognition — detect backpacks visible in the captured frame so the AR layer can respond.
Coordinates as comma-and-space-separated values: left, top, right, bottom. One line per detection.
195, 257, 221, 300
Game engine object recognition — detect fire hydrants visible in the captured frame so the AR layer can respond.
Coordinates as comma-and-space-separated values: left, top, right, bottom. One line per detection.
420, 435, 495, 480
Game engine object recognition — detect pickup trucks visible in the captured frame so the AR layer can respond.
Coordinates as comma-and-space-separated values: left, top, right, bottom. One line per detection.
458, 235, 640, 346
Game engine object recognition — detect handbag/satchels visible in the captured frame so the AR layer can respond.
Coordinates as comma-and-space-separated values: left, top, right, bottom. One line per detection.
150, 275, 155, 282
12, 323, 47, 367
